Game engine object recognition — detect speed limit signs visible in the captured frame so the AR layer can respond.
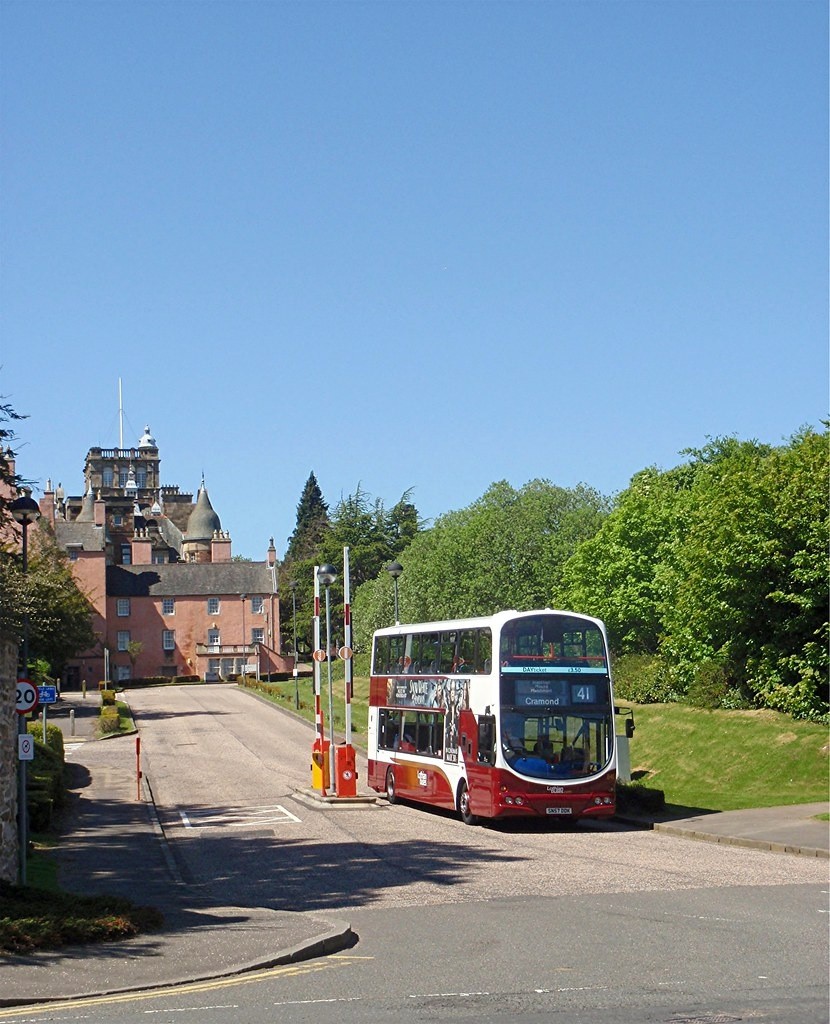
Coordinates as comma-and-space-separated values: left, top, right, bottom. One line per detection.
16, 678, 40, 714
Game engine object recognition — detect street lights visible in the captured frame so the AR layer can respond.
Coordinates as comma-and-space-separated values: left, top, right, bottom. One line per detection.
317, 562, 338, 798
387, 563, 404, 659
9, 494, 42, 887
239, 593, 248, 686
289, 580, 300, 709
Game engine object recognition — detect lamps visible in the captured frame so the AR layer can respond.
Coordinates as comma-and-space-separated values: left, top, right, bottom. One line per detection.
213, 622, 216, 628
254, 646, 258, 656
187, 657, 192, 664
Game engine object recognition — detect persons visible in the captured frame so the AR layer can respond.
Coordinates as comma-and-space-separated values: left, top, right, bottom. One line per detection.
541, 742, 560, 764
395, 726, 416, 747
533, 734, 543, 756
502, 726, 522, 760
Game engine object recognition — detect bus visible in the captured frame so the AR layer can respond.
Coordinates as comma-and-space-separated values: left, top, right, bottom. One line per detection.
364, 608, 637, 826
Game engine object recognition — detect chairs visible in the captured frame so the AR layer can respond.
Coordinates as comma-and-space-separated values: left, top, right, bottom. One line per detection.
413, 661, 419, 674
483, 658, 492, 674
430, 660, 436, 673
377, 663, 383, 674
561, 747, 585, 770
533, 742, 553, 755
393, 733, 416, 751
384, 663, 393, 674
459, 665, 475, 673
443, 663, 452, 673
